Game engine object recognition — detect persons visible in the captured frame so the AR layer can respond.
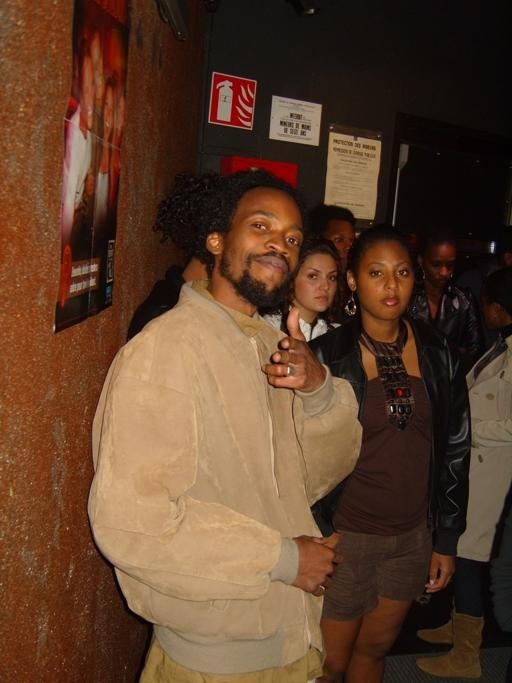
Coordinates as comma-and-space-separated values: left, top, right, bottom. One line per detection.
126, 172, 229, 341
66, 27, 125, 245
84, 170, 365, 681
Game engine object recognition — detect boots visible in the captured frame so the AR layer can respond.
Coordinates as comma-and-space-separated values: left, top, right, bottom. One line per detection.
415, 613, 485, 679
416, 597, 456, 643
